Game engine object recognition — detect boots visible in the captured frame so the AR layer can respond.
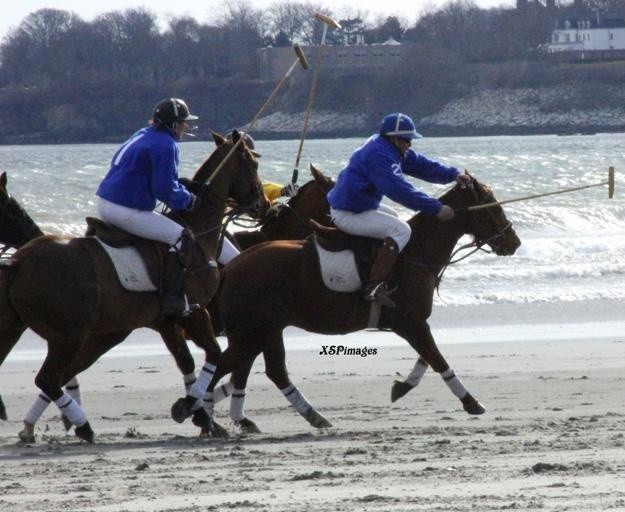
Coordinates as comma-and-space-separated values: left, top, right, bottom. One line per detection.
160, 255, 200, 315
367, 237, 396, 307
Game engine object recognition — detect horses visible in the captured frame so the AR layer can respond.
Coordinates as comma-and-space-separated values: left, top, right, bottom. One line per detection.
0, 127, 272, 444
217, 168, 522, 439
180, 162, 337, 440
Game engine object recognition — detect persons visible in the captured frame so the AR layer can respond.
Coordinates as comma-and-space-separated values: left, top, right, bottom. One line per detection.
324, 112, 474, 311
93, 95, 205, 315
210, 128, 302, 268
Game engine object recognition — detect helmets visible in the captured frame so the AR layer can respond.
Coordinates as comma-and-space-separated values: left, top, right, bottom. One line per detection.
380, 112, 423, 139
152, 98, 200, 136
